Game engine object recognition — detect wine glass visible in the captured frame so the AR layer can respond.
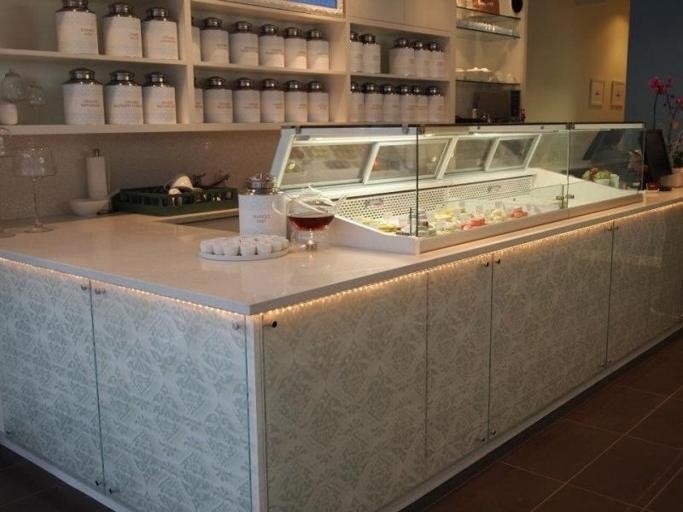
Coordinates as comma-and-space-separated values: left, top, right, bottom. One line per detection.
1, 68, 56, 238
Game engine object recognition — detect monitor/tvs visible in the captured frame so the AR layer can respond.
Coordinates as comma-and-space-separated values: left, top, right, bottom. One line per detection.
581, 130, 626, 178
637, 129, 673, 191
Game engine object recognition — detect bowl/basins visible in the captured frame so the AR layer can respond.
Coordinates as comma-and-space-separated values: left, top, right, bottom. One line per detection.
69, 200, 107, 218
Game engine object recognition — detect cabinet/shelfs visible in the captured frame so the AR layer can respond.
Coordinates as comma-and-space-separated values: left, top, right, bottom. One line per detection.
0, 3, 530, 128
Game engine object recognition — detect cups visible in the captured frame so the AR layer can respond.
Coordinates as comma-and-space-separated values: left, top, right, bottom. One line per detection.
457, 66, 492, 83
201, 234, 288, 256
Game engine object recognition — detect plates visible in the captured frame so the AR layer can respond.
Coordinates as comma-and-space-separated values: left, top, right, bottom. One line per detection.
198, 251, 287, 261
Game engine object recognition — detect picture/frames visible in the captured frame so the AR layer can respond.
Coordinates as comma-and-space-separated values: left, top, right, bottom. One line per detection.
589, 77, 625, 110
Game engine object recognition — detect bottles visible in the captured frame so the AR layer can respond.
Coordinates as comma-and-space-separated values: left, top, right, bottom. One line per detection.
194, 66, 332, 122
60, 62, 187, 124
192, 14, 331, 74
238, 172, 287, 238
350, 31, 382, 76
391, 38, 447, 80
85, 149, 110, 198
53, 0, 183, 61
349, 77, 447, 122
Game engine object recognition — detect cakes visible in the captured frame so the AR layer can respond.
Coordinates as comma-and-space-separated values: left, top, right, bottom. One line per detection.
200, 234, 289, 256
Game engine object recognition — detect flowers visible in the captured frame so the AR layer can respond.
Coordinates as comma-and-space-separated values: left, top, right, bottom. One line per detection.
650, 73, 682, 142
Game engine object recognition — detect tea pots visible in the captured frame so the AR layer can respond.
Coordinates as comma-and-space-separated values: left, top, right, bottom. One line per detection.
271, 185, 348, 233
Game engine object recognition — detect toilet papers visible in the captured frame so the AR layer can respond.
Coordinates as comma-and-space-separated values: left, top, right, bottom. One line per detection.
85, 156, 112, 216
